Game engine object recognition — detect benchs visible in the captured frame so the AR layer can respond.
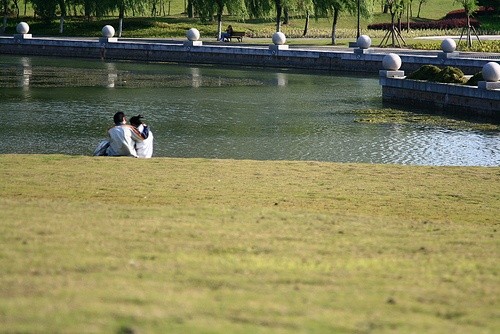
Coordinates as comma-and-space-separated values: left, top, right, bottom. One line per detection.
222, 32, 245, 42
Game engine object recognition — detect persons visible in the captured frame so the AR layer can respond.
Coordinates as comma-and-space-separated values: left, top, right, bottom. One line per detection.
220, 25, 233, 42
129, 115, 155, 158
95, 112, 149, 157
